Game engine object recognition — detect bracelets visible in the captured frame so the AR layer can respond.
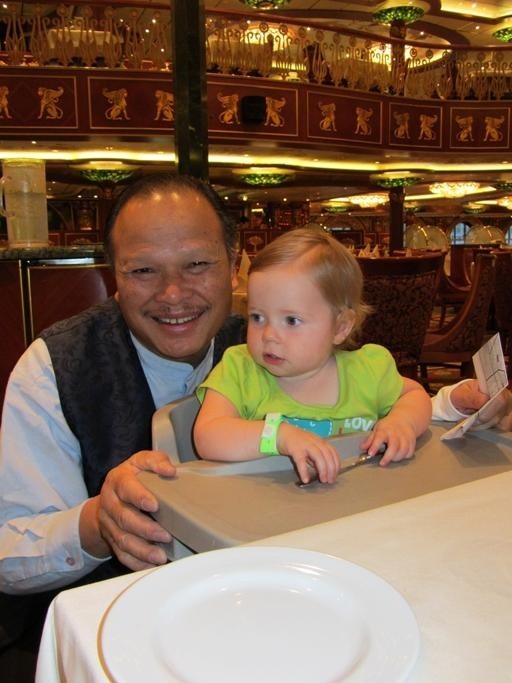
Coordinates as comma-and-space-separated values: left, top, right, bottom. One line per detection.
259, 412, 285, 455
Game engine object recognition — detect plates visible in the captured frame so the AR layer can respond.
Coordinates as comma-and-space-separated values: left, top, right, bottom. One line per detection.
97, 544, 423, 683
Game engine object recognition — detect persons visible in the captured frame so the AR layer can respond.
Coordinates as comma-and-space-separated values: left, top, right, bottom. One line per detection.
0, 169, 512, 655
191, 225, 434, 484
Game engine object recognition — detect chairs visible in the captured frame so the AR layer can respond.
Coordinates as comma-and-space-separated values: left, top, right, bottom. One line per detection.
154, 392, 206, 464
356, 243, 511, 385
